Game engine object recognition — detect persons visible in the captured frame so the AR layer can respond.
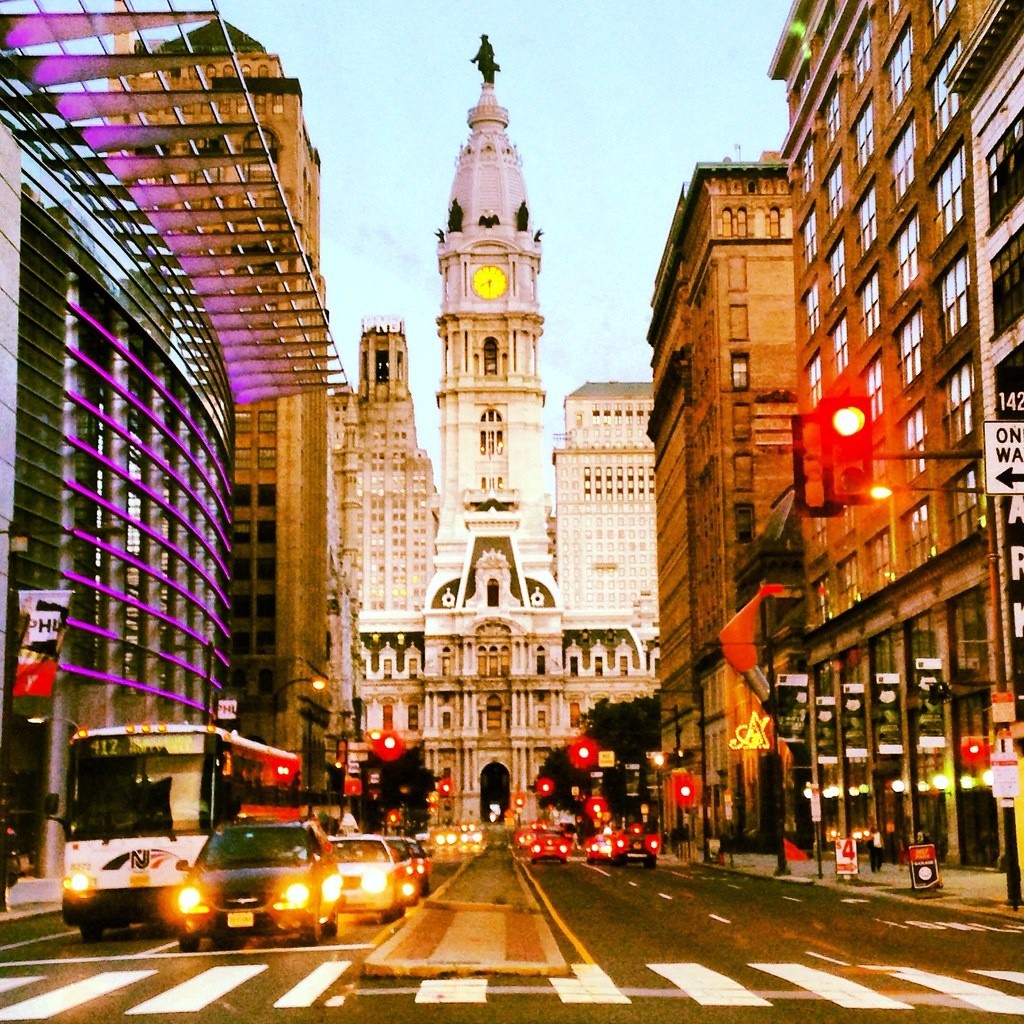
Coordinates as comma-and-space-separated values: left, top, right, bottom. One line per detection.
603, 820, 613, 834
518, 201, 529, 231
560, 823, 581, 849
866, 824, 885, 871
470, 34, 500, 83
646, 814, 659, 832
448, 198, 463, 233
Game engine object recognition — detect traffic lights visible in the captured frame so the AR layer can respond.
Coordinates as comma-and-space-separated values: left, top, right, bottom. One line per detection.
824, 402, 873, 504
679, 785, 692, 802
540, 780, 551, 796
382, 735, 395, 755
440, 782, 450, 796
577, 746, 590, 762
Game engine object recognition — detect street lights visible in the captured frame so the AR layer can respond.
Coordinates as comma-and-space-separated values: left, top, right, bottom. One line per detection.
249, 676, 326, 740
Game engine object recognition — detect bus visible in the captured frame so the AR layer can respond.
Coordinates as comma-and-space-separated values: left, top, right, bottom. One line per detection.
43, 723, 301, 941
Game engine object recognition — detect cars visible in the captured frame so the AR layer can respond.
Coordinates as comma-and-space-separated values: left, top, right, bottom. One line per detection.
435, 828, 457, 846
385, 836, 431, 906
531, 834, 568, 863
587, 834, 618, 863
328, 833, 404, 920
613, 825, 658, 868
462, 830, 482, 843
175, 820, 342, 954
520, 834, 535, 850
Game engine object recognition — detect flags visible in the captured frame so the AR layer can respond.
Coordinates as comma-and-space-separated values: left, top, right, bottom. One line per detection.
13, 589, 75, 695
